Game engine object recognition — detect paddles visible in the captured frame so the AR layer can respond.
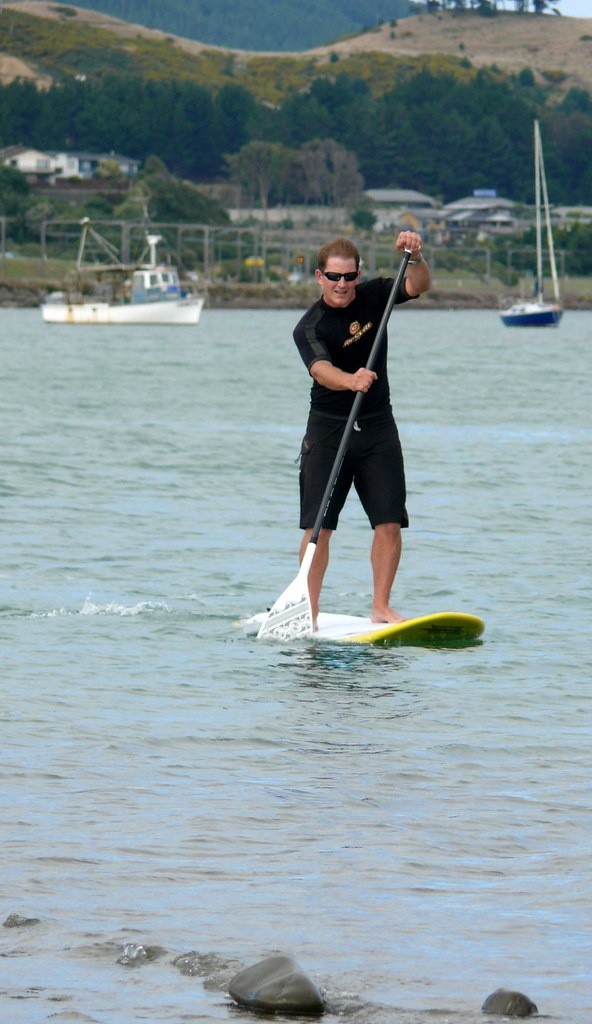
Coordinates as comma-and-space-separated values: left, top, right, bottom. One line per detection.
256, 243, 413, 646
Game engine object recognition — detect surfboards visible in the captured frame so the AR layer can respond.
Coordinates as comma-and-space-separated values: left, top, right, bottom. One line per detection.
236, 604, 487, 650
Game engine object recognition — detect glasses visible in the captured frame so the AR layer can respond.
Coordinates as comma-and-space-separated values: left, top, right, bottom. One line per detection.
322, 263, 360, 282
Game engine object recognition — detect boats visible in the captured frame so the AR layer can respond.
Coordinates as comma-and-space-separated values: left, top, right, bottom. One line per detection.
40, 266, 205, 325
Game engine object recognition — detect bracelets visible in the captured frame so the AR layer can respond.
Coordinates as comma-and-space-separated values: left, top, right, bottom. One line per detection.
407, 252, 423, 265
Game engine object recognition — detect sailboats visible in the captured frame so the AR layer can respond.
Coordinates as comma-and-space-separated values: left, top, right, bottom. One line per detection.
497, 119, 564, 327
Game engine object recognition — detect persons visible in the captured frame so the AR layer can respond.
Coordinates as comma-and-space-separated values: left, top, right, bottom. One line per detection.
293, 230, 432, 630
